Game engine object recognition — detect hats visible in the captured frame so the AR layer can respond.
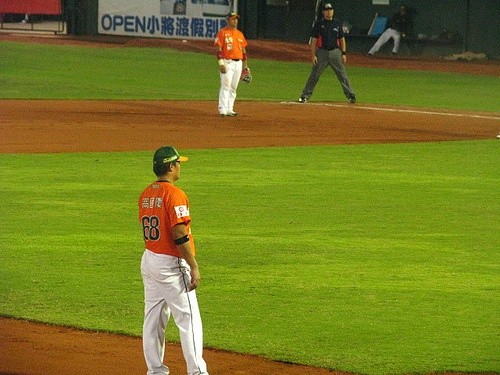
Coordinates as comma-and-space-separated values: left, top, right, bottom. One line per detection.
153, 146, 188, 164
320, 2, 335, 11
226, 11, 240, 20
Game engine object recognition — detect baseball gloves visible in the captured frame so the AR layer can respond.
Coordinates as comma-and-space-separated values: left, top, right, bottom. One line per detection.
239, 68, 252, 83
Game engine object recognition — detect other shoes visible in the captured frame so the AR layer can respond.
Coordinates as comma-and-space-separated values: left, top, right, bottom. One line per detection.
299, 97, 306, 103
349, 97, 355, 103
227, 111, 236, 116
220, 111, 227, 114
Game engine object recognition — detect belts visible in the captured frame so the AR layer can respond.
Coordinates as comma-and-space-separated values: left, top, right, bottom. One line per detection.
232, 59, 239, 61
321, 48, 334, 50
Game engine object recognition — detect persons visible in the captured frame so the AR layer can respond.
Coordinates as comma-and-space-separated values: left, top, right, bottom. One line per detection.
298, 2, 356, 103
138, 146, 209, 375
368, 4, 412, 55
212, 11, 250, 116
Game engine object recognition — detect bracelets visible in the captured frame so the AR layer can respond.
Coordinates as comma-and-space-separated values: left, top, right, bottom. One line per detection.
342, 52, 346, 55
